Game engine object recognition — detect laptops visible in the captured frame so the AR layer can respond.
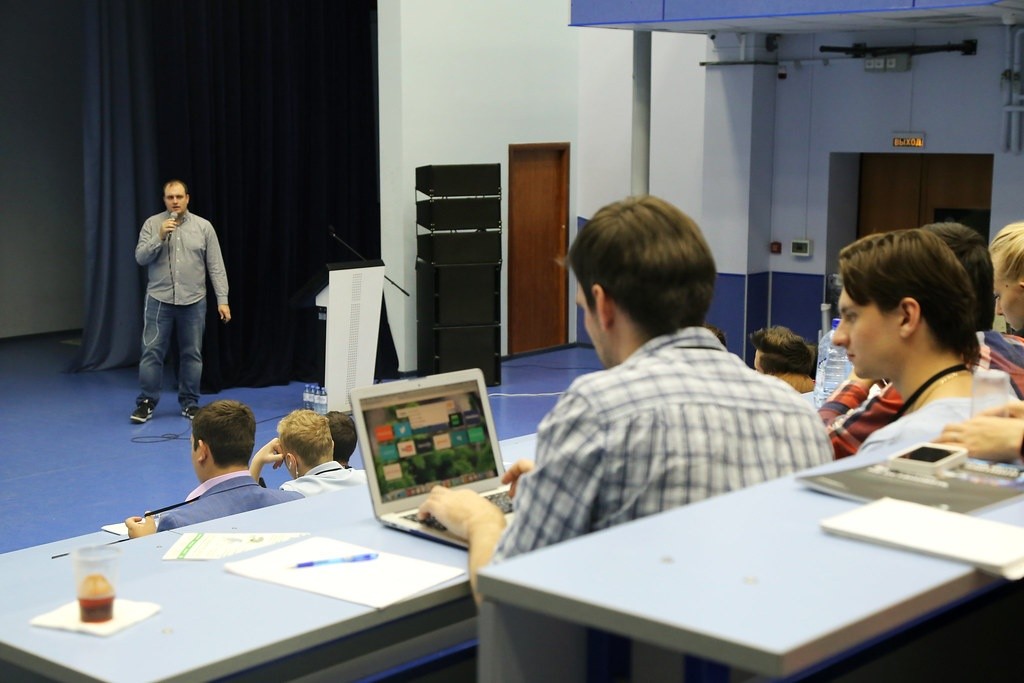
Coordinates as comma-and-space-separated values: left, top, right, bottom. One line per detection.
347, 367, 522, 549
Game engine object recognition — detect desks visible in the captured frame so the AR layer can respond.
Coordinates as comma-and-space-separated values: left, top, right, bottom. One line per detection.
0, 517, 159, 567
476, 436, 1024, 683
0, 434, 538, 683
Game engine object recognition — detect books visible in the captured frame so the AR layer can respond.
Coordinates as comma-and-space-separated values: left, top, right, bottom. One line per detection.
797, 464, 1024, 582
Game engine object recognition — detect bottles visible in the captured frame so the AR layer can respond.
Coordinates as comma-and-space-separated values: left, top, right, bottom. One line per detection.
814, 317, 846, 410
303, 384, 309, 409
313, 386, 321, 413
308, 385, 315, 411
319, 387, 328, 415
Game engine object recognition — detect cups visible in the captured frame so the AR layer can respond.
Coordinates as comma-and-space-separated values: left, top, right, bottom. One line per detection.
70, 544, 123, 624
969, 370, 1009, 419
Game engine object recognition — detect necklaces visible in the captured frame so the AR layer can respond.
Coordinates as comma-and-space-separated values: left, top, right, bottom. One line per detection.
914, 371, 970, 411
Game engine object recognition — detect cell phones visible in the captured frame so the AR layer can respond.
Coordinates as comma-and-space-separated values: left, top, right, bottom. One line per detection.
887, 442, 968, 477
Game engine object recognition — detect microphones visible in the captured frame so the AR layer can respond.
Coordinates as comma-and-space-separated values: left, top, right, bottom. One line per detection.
169, 211, 179, 236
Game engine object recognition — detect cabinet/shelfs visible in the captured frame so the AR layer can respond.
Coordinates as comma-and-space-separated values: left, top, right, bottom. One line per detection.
415, 162, 503, 265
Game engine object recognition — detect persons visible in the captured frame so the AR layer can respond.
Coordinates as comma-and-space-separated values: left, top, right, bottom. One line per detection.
130, 179, 232, 423
704, 222, 1024, 466
125, 399, 304, 544
249, 410, 368, 501
417, 194, 835, 611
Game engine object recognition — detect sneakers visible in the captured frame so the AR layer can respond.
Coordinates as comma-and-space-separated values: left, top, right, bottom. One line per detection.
182, 406, 199, 420
130, 398, 153, 424
